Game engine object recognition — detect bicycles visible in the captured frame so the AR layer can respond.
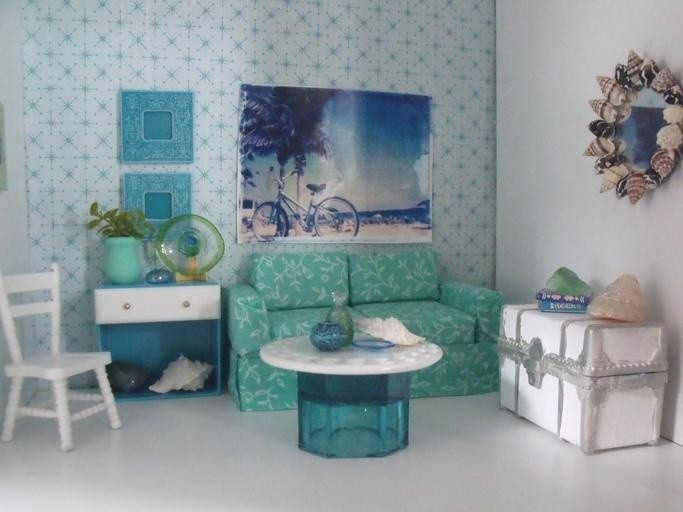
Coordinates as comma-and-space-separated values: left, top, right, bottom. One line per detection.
249, 165, 362, 239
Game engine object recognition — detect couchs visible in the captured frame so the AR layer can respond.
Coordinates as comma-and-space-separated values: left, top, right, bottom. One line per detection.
222, 249, 504, 412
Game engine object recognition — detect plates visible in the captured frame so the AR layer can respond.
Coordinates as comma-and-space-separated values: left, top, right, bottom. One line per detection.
350, 338, 395, 350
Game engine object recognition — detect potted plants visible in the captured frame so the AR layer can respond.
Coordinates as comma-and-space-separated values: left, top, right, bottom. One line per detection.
89, 202, 154, 286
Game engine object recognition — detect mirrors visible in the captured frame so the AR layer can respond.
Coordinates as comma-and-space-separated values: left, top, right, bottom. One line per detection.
583, 50, 683, 206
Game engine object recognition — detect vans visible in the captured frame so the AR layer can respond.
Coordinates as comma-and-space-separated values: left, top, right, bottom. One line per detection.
238, 194, 267, 228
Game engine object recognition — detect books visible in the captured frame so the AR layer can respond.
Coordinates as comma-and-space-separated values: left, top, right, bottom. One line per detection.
538, 296, 589, 314
532, 286, 589, 305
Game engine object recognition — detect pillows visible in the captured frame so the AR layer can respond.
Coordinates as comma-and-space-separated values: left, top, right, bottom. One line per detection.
249, 250, 440, 311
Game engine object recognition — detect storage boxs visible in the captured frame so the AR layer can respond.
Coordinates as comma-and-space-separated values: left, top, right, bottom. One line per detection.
498, 304, 669, 455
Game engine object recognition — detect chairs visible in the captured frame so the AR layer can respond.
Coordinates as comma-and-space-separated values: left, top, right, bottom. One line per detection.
0, 263, 123, 451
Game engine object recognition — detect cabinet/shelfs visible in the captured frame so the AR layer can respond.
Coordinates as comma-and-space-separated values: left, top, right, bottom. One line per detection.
94, 280, 222, 402
